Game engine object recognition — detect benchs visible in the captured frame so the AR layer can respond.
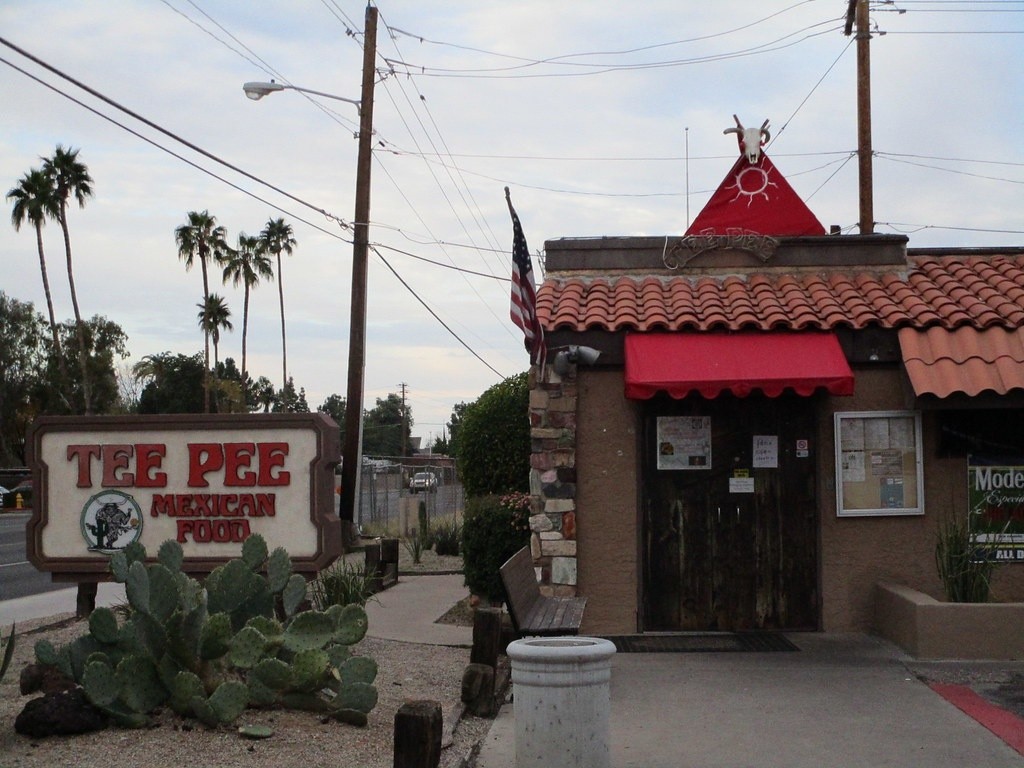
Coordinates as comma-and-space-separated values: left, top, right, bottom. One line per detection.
497, 546, 587, 636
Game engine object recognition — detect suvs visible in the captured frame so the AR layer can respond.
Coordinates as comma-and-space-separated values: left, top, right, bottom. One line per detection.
409, 472, 439, 495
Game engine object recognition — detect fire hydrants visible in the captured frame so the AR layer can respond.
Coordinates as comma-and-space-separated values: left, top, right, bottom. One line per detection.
16, 492, 24, 511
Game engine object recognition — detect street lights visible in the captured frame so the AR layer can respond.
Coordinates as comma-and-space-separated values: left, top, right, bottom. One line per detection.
241, 80, 374, 525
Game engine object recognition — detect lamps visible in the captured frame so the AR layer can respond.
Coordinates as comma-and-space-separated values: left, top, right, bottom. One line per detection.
553, 342, 602, 374
